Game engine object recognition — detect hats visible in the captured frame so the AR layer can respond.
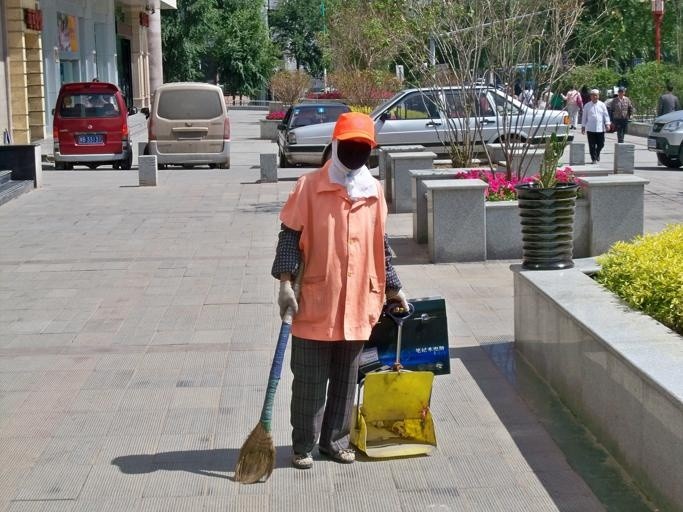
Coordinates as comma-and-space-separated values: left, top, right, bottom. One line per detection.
590, 89, 600, 95
333, 112, 378, 148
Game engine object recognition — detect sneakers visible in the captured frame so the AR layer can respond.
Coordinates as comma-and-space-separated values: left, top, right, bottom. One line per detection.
292, 451, 313, 468
319, 445, 357, 463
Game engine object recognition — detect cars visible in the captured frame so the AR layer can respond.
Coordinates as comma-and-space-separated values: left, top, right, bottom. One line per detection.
281, 83, 571, 171
645, 109, 683, 170
274, 102, 353, 168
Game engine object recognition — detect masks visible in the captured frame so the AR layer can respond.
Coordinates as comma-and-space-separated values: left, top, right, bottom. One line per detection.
337, 140, 370, 169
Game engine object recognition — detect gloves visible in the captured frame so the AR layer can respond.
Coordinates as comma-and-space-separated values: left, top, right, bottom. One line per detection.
278, 281, 299, 318
386, 289, 409, 313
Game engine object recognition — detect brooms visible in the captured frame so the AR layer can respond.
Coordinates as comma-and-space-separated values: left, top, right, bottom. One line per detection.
232, 263, 305, 485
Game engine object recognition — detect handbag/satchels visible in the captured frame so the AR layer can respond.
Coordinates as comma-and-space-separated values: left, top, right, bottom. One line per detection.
577, 95, 583, 110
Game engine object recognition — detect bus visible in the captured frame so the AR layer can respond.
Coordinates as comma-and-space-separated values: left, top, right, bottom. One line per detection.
491, 62, 550, 92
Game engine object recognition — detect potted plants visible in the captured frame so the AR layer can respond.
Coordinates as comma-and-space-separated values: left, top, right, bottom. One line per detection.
511, 134, 584, 270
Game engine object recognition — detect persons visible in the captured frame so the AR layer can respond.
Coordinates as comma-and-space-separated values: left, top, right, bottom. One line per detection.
85, 78, 119, 114
269, 111, 410, 470
579, 88, 611, 165
495, 78, 681, 144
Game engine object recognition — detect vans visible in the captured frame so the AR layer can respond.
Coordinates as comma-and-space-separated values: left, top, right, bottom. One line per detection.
50, 80, 138, 172
139, 81, 234, 170
